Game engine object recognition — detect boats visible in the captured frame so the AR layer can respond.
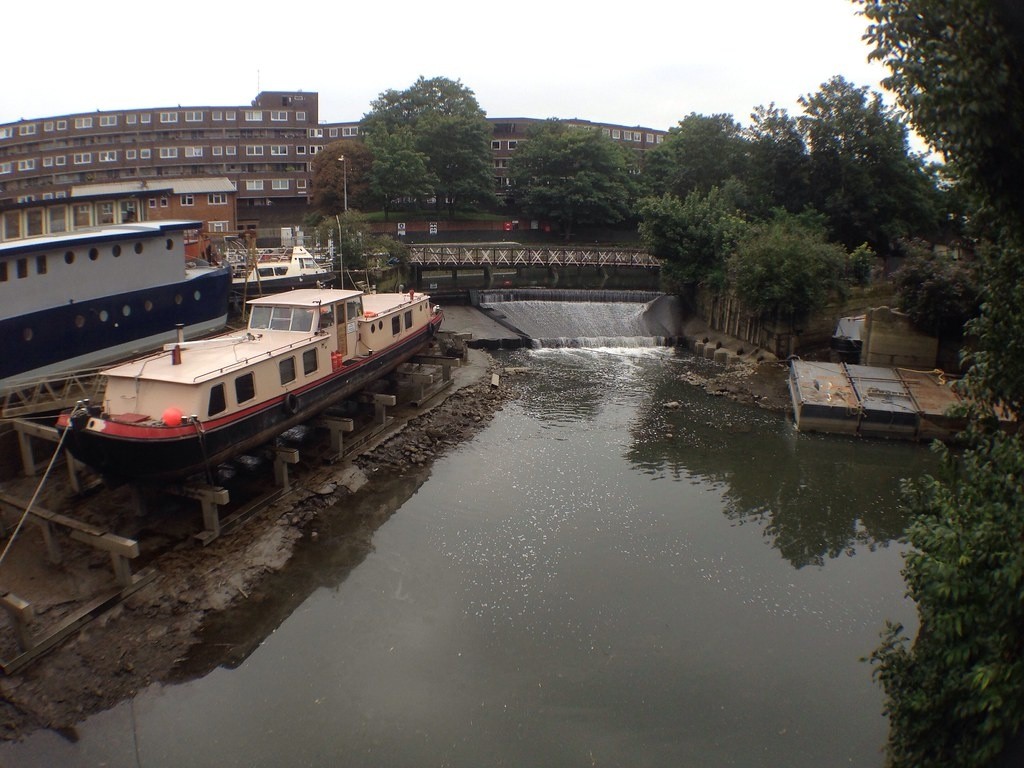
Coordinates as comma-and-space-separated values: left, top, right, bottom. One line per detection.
57, 285, 444, 483
788, 355, 1024, 444
230, 245, 338, 289
830, 314, 866, 353
0, 219, 231, 397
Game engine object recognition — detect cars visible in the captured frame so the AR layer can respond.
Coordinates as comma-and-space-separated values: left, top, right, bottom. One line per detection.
391, 192, 456, 204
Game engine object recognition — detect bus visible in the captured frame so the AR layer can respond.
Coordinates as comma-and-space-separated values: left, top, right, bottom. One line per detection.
360, 252, 391, 262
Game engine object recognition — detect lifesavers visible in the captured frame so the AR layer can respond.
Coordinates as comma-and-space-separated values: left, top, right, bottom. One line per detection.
364, 311, 376, 318
310, 308, 328, 312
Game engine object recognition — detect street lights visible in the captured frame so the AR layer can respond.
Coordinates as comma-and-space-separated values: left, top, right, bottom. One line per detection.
338, 154, 348, 212
